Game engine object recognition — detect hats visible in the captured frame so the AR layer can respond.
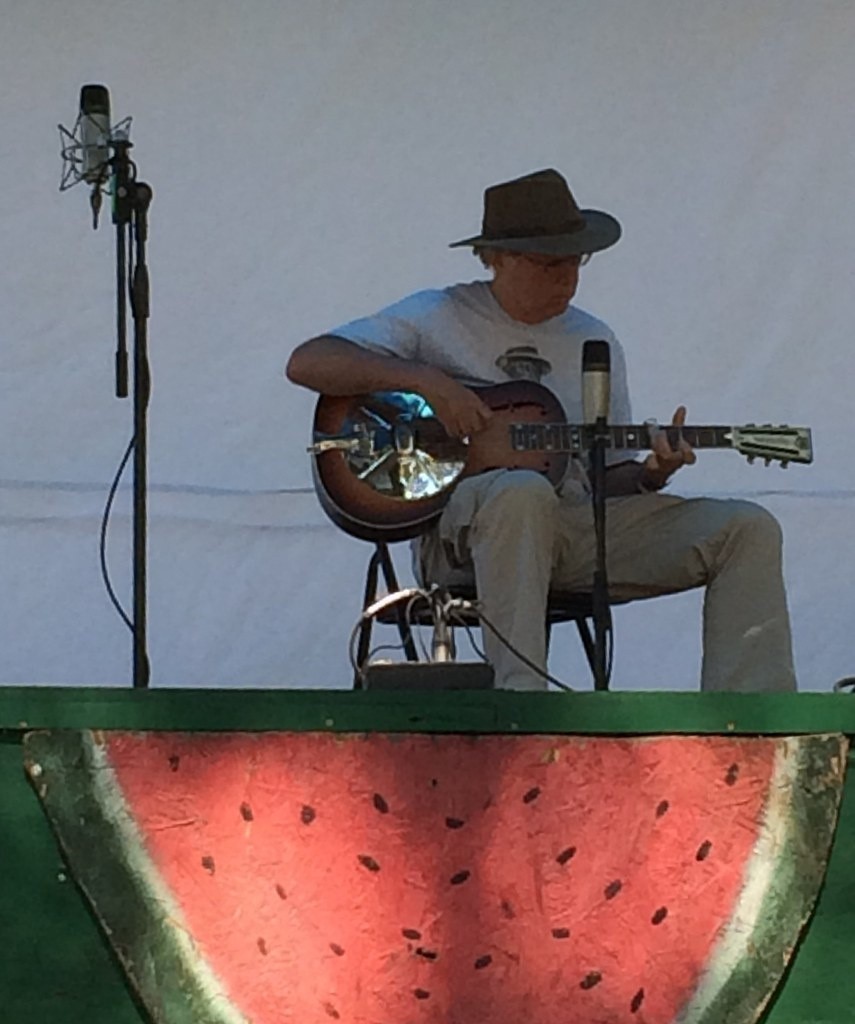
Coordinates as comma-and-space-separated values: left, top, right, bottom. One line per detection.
447, 170, 623, 259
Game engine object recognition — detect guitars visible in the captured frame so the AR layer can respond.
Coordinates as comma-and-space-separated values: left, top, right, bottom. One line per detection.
310, 378, 813, 544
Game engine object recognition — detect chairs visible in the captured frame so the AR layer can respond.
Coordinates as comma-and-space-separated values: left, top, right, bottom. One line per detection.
351, 542, 618, 687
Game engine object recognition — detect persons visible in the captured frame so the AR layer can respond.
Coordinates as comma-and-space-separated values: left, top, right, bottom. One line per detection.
285, 170, 797, 691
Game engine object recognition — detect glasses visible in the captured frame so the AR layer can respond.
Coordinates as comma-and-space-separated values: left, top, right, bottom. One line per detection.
517, 252, 594, 268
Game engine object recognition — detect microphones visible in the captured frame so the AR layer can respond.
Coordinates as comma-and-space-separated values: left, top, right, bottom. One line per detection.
582, 339, 612, 461
80, 83, 110, 229
